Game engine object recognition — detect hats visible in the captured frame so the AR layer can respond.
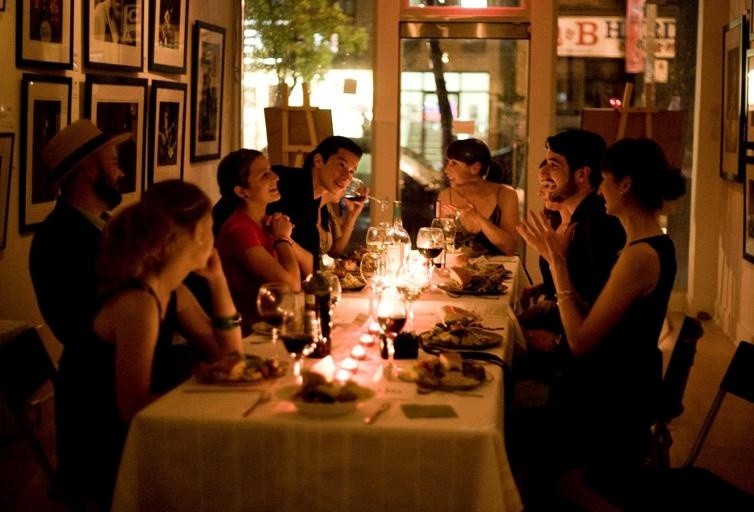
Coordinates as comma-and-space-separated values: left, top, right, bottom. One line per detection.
41, 116, 131, 184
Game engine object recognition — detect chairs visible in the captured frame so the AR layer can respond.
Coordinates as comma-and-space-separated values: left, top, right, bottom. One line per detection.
563, 341, 754, 511
0, 327, 110, 511
515, 314, 706, 511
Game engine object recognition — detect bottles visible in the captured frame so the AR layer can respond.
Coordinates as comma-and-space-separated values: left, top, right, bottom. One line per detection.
303, 247, 331, 360
391, 200, 404, 227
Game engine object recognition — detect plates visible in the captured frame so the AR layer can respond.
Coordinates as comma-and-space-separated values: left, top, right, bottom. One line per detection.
396, 271, 513, 388
272, 382, 375, 416
339, 280, 366, 291
199, 359, 284, 384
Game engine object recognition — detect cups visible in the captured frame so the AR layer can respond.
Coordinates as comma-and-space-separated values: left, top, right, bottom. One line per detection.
306, 273, 342, 307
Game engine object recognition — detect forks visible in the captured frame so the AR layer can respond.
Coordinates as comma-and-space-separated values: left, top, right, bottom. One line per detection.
242, 392, 270, 416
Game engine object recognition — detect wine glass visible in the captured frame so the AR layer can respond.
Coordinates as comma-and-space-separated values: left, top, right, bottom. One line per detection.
341, 177, 392, 213
360, 218, 456, 383
256, 282, 317, 388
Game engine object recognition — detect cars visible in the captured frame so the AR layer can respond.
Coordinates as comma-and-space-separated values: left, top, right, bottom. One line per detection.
257, 136, 525, 259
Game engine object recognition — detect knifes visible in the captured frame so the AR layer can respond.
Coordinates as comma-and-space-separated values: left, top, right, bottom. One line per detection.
365, 402, 391, 423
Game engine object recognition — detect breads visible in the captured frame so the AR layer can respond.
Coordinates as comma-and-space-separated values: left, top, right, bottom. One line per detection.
300, 355, 337, 397
448, 266, 471, 287
210, 357, 247, 377
436, 328, 475, 345
439, 352, 463, 380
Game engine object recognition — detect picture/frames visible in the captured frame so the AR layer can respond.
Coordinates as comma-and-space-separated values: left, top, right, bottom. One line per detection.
748, 0, 754, 40
719, 14, 747, 181
18, 73, 72, 236
147, 0, 190, 74
742, 156, 754, 266
17, 0, 75, 71
83, 74, 148, 216
83, 0, 145, 73
745, 47, 754, 151
0, 131, 15, 250
191, 19, 227, 164
147, 79, 188, 190
